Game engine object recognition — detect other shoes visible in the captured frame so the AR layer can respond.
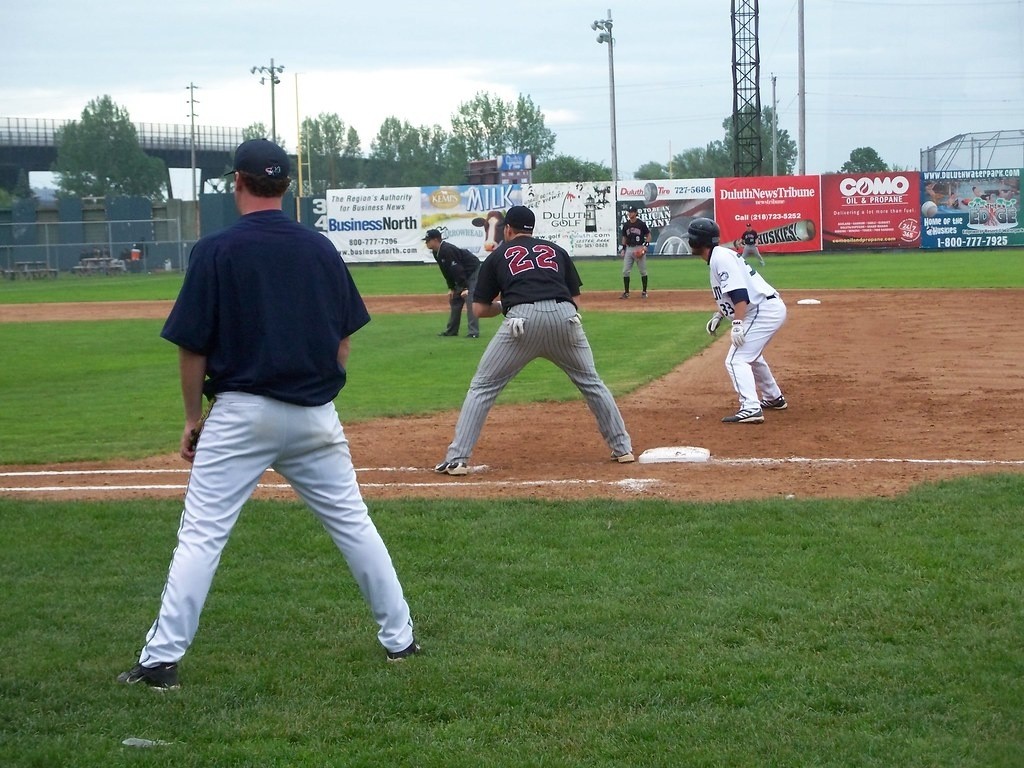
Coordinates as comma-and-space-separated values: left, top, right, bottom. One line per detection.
759, 260, 765, 267
464, 333, 480, 338
437, 331, 457, 336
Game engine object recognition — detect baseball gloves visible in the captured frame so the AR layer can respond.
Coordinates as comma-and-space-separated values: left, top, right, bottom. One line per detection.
633, 248, 646, 259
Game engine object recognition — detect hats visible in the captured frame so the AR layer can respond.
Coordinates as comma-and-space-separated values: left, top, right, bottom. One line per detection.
496, 204, 536, 231
223, 138, 290, 179
747, 223, 751, 227
421, 229, 441, 240
625, 205, 637, 212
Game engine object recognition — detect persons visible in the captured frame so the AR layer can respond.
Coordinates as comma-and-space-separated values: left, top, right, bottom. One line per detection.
925, 181, 1014, 208
422, 230, 480, 338
618, 205, 652, 299
741, 223, 764, 266
78, 238, 147, 273
118, 137, 419, 693
681, 218, 787, 424
429, 206, 634, 473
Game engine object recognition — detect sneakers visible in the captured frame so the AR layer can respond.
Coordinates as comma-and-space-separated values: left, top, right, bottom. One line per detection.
116, 648, 182, 692
721, 408, 765, 424
384, 629, 421, 663
619, 291, 631, 299
641, 291, 648, 298
759, 395, 787, 409
435, 460, 470, 476
610, 450, 635, 463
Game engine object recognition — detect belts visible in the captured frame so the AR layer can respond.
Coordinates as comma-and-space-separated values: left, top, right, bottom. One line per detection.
518, 298, 572, 304
766, 294, 776, 300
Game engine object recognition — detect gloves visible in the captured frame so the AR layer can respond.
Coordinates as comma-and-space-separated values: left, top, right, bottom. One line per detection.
568, 312, 582, 324
706, 312, 725, 336
730, 319, 746, 349
506, 317, 525, 338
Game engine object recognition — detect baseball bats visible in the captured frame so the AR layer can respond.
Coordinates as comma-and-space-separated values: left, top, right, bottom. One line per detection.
719, 219, 816, 249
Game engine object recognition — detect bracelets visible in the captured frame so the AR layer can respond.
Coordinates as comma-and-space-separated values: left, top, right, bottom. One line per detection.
497, 300, 501, 307
644, 241, 649, 247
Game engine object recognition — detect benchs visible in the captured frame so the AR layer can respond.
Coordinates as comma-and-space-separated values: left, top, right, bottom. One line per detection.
73, 266, 122, 277
4, 268, 57, 278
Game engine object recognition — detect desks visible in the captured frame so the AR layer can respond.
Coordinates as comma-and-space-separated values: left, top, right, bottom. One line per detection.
83, 259, 114, 266
15, 261, 45, 264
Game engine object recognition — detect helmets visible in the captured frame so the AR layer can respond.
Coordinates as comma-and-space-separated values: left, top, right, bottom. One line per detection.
679, 217, 721, 248
